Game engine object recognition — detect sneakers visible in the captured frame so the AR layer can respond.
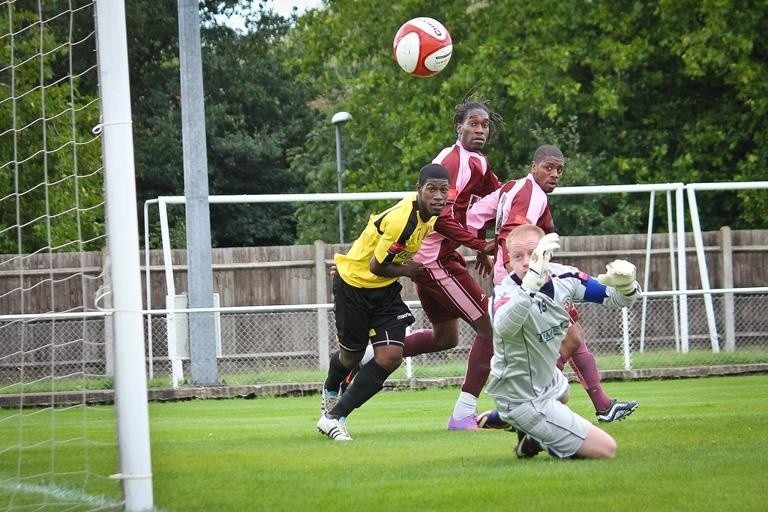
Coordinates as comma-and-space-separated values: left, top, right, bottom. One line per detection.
319, 380, 341, 415
446, 411, 488, 431
593, 397, 639, 424
515, 432, 545, 459
316, 412, 355, 441
476, 409, 517, 432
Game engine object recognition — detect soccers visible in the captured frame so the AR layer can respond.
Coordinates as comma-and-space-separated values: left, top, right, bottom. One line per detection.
393, 17, 454, 78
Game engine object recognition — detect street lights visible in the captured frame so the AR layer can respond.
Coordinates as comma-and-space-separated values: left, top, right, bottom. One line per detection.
331, 112, 352, 243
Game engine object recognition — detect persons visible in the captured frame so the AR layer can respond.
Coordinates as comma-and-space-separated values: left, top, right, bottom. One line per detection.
315, 162, 451, 444
476, 220, 645, 460
336, 76, 503, 432
463, 143, 640, 425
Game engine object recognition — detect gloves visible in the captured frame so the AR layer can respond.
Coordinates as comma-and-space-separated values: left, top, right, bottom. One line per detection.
596, 259, 640, 298
520, 230, 561, 294
